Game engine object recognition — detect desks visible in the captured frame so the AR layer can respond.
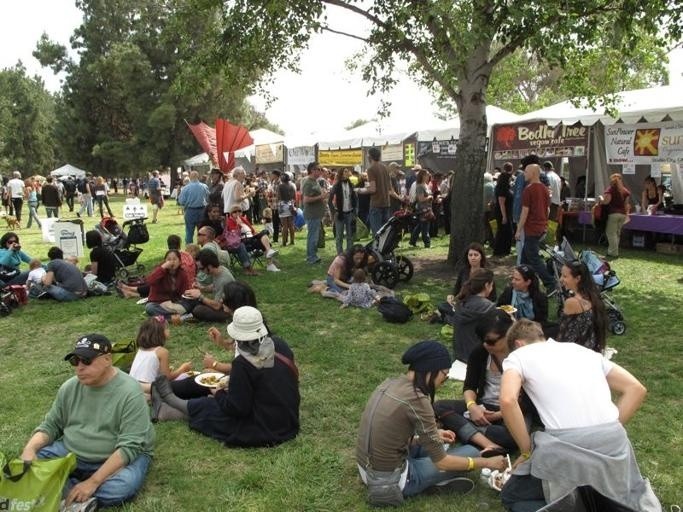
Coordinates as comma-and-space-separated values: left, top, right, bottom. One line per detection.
575, 209, 683, 258
557, 207, 593, 247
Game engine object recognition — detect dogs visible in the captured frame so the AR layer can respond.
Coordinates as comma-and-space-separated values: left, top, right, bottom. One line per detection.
2, 214, 19, 230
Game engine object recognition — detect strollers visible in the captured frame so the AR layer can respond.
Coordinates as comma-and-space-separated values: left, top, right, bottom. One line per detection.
0, 262, 21, 317
361, 206, 418, 286
91, 214, 149, 276
538, 236, 628, 338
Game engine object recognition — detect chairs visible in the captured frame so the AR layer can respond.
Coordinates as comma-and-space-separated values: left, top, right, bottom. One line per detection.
248, 247, 266, 274
193, 217, 246, 273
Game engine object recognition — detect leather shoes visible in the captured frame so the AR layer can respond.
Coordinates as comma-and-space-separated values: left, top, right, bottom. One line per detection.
149, 374, 174, 423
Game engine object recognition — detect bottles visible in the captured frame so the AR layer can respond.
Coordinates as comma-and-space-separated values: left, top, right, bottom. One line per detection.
475, 468, 493, 510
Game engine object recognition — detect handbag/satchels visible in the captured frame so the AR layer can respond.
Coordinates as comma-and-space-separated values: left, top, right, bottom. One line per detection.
356, 218, 367, 241
403, 293, 434, 314
366, 469, 403, 508
418, 208, 436, 222
378, 296, 413, 324
0, 451, 78, 512
127, 224, 150, 244
112, 340, 135, 370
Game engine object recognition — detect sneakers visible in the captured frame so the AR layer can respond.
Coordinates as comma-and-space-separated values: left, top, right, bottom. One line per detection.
265, 248, 279, 259
244, 266, 256, 275
267, 263, 280, 272
423, 476, 474, 496
59, 497, 97, 512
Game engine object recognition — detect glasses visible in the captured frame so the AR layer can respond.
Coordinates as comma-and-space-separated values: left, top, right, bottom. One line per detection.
70, 356, 92, 366
480, 334, 503, 346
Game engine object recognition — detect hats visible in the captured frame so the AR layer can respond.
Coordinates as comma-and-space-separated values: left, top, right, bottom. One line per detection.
402, 340, 451, 372
65, 333, 112, 359
227, 305, 268, 341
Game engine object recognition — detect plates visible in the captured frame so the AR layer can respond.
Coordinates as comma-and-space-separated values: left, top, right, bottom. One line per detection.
494, 305, 518, 315
194, 372, 226, 388
180, 292, 193, 298
488, 469, 512, 491
